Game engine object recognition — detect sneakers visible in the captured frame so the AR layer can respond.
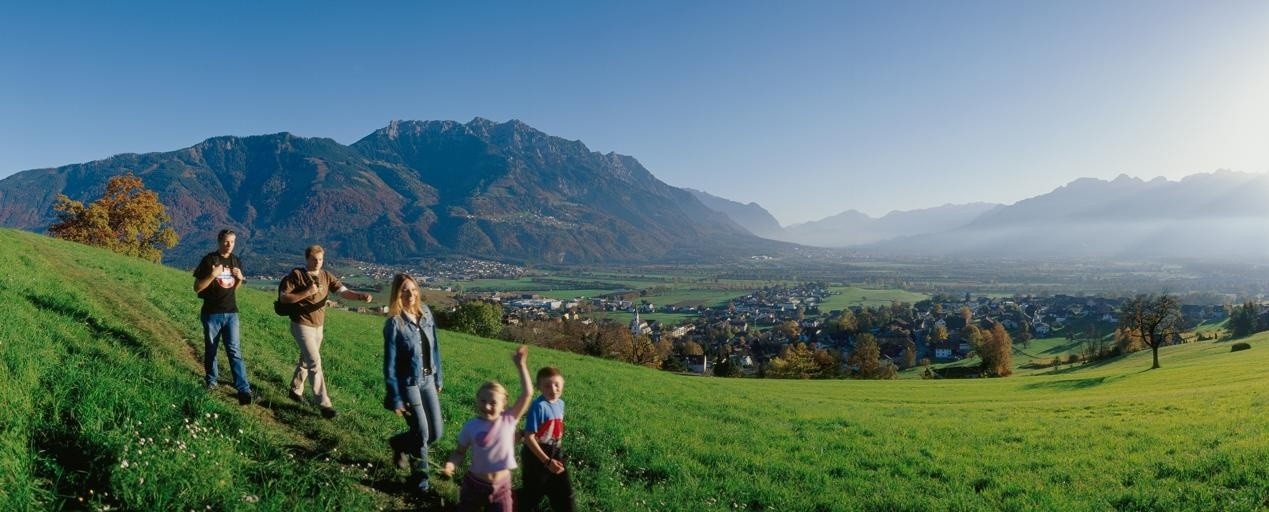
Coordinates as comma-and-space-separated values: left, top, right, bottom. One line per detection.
322, 407, 336, 417
289, 390, 306, 404
241, 389, 255, 398
390, 437, 408, 470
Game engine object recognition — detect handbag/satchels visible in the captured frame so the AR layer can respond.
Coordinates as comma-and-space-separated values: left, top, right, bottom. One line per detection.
274, 267, 311, 316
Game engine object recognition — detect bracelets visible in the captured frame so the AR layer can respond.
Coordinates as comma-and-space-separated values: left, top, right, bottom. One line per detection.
542, 456, 552, 469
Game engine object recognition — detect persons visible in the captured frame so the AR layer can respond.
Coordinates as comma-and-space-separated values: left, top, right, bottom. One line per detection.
277, 244, 373, 421
380, 274, 445, 499
510, 365, 580, 511
443, 346, 533, 511
191, 228, 253, 403
